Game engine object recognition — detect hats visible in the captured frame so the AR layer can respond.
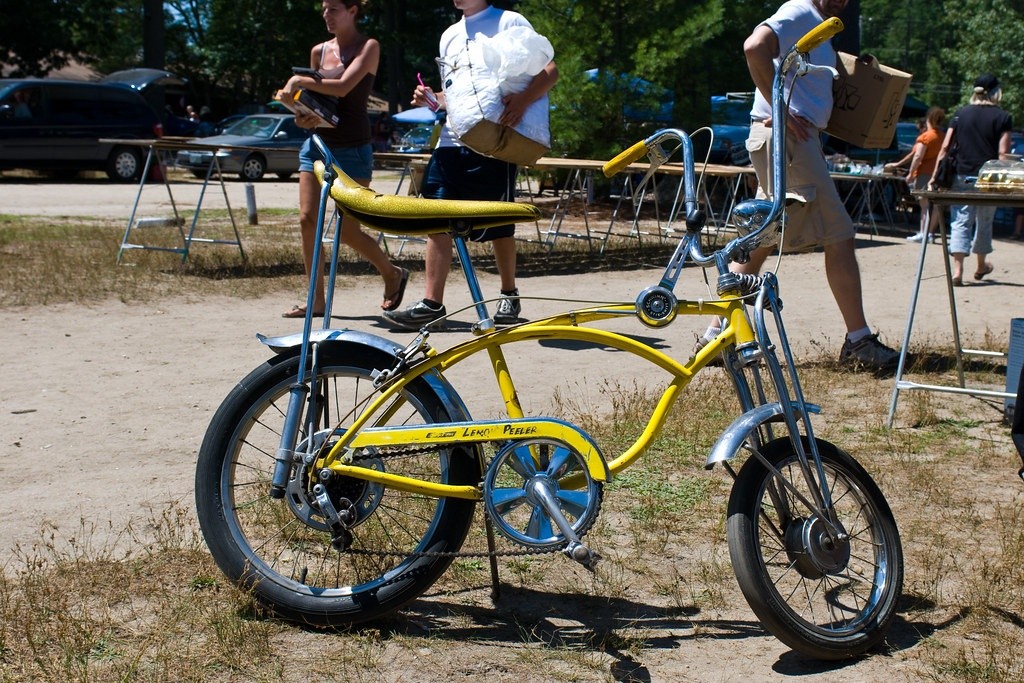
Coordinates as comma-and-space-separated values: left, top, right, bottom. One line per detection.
973, 73, 1000, 95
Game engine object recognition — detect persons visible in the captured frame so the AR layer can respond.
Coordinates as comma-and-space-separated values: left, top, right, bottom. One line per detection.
885, 106, 947, 243
382, 0, 559, 332
279, 0, 409, 318
689, 0, 899, 369
430, 118, 446, 148
183, 105, 212, 123
2, 94, 32, 117
371, 125, 395, 152
929, 74, 1012, 285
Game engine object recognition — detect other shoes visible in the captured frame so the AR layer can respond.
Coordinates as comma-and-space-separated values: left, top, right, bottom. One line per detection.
927, 233, 933, 243
906, 232, 923, 243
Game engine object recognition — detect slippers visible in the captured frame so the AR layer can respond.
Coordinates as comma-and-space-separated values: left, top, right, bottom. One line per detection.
282, 304, 326, 318
952, 278, 964, 286
383, 268, 409, 311
975, 263, 993, 281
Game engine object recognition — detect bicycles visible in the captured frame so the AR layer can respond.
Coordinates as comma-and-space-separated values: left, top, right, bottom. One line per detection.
195, 16, 905, 662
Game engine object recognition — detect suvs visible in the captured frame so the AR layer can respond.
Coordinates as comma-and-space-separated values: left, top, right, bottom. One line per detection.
0, 68, 191, 185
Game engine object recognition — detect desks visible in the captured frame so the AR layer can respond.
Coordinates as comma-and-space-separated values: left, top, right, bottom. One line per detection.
97, 135, 304, 264
372, 152, 1024, 430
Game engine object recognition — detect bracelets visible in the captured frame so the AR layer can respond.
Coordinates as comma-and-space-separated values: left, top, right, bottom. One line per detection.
429, 94, 437, 110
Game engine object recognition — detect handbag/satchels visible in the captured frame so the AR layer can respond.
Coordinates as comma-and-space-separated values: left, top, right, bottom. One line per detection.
935, 129, 956, 190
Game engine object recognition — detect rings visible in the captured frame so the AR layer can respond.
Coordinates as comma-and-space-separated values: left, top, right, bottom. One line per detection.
302, 118, 304, 121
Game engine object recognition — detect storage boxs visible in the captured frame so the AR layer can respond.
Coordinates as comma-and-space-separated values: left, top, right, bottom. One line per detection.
821, 50, 914, 150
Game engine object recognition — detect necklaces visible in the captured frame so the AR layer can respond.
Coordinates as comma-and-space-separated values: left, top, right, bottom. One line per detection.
333, 42, 349, 60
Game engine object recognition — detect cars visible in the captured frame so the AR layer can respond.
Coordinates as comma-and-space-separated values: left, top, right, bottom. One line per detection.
174, 112, 310, 182
396, 125, 434, 154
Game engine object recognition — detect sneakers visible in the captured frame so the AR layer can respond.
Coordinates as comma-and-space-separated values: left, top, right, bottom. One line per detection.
381, 300, 448, 332
689, 333, 725, 366
493, 288, 521, 324
838, 332, 910, 376
